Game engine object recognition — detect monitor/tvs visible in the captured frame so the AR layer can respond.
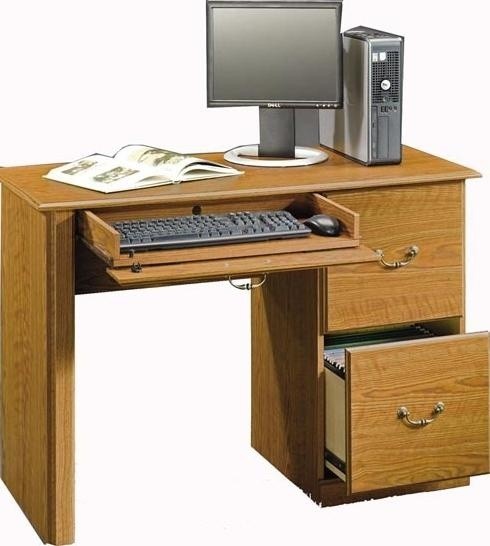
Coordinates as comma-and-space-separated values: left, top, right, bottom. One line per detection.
205, 0, 342, 166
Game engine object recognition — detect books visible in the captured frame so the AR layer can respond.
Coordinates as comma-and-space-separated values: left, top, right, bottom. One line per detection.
41, 142, 248, 194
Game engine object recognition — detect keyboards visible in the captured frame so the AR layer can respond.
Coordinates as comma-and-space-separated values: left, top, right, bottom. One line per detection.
109, 210, 312, 254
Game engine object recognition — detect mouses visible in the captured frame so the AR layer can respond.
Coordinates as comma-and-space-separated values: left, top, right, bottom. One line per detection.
303, 214, 342, 237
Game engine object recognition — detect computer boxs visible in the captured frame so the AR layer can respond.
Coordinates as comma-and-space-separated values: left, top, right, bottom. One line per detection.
319, 25, 403, 166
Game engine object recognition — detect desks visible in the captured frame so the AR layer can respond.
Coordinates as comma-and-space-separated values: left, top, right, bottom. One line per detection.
0, 142, 490, 546
